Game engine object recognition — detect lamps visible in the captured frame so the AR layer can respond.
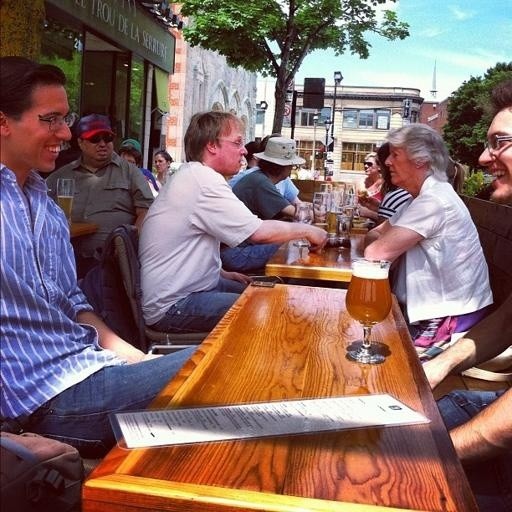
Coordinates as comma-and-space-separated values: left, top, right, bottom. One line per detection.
140, 0, 186, 33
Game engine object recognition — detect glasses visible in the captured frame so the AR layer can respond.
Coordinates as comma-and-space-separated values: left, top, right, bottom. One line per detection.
39, 113, 75, 131
483, 134, 512, 153
89, 136, 113, 144
364, 162, 373, 166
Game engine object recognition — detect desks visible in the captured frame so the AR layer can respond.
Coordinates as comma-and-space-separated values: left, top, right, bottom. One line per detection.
68, 219, 99, 240
74, 277, 480, 512
264, 213, 386, 287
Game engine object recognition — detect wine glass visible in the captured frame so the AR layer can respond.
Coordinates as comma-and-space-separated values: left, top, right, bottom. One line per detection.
295, 182, 358, 250
343, 256, 391, 364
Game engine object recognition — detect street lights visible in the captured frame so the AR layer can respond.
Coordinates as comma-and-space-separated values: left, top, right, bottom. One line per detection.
309, 112, 319, 179
322, 115, 331, 180
260, 100, 268, 140
330, 69, 343, 151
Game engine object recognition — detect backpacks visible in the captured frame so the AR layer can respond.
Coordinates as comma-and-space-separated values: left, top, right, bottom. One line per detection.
80, 225, 148, 352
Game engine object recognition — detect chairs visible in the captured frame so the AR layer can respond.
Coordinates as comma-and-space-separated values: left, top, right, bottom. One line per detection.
106, 222, 221, 358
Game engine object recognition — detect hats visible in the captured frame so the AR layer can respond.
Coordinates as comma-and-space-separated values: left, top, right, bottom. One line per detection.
252, 137, 307, 166
79, 113, 116, 138
120, 139, 142, 153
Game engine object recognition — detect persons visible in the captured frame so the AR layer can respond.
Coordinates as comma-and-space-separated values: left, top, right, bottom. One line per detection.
355, 142, 414, 221
357, 149, 382, 205
45, 110, 173, 272
140, 110, 329, 330
1, 55, 198, 458
424, 82, 512, 456
363, 123, 496, 363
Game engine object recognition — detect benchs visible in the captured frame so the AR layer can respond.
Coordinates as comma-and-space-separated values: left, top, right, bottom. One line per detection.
389, 187, 510, 510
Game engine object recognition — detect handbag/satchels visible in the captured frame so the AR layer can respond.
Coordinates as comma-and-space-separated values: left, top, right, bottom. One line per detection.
451, 330, 512, 382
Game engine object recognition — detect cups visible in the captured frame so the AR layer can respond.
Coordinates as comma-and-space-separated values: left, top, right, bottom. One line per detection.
56, 176, 76, 226
